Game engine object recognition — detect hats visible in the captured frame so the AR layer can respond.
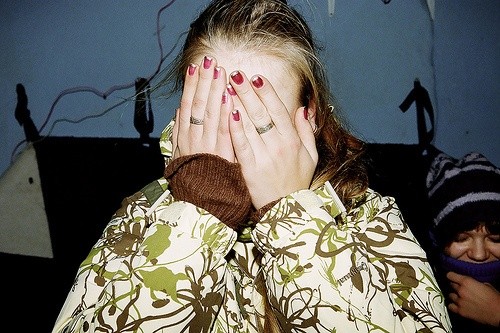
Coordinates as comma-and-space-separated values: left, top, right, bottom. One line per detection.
425, 151, 500, 245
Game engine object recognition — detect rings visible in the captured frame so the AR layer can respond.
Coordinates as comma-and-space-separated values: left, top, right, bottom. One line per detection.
256, 121, 275, 134
189, 115, 203, 125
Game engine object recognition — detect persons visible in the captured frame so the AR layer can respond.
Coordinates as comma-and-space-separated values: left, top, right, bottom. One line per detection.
413, 151, 500, 333
45, 0, 454, 333
